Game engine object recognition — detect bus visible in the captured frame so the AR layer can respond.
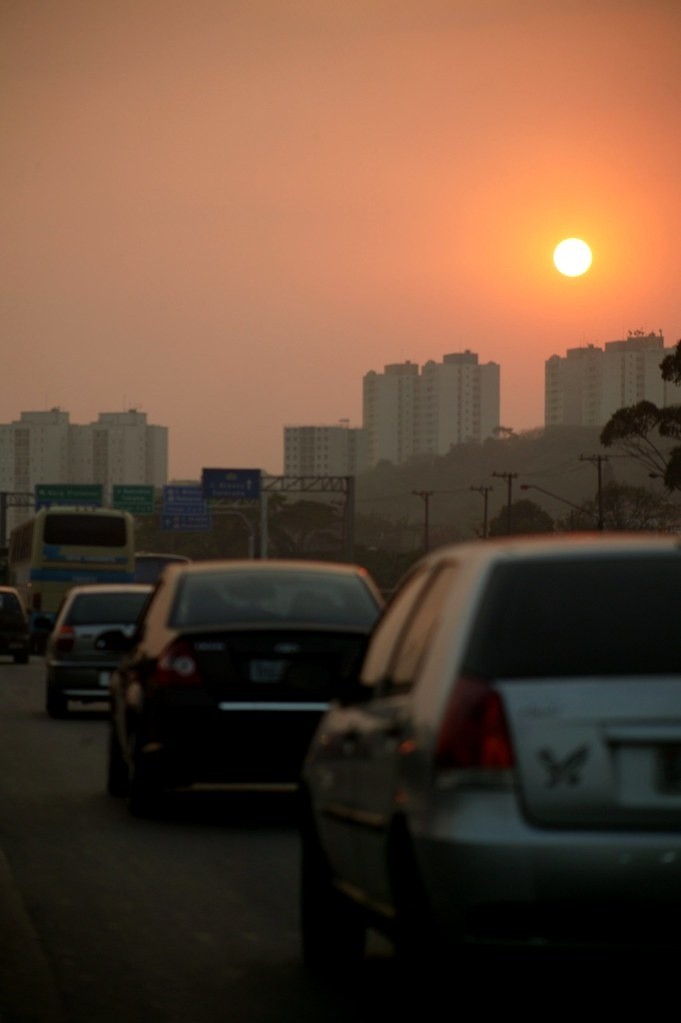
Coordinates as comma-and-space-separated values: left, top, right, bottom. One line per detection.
10, 507, 137, 656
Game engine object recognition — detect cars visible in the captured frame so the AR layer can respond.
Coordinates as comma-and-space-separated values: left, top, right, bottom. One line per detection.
96, 561, 389, 816
1, 588, 35, 665
295, 530, 681, 979
35, 581, 159, 720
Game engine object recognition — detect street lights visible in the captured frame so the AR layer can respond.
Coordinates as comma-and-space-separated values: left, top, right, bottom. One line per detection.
519, 481, 605, 529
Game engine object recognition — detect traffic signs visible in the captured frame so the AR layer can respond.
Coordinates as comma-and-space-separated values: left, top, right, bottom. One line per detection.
32, 485, 104, 514
162, 483, 212, 532
112, 482, 156, 516
201, 466, 262, 503
